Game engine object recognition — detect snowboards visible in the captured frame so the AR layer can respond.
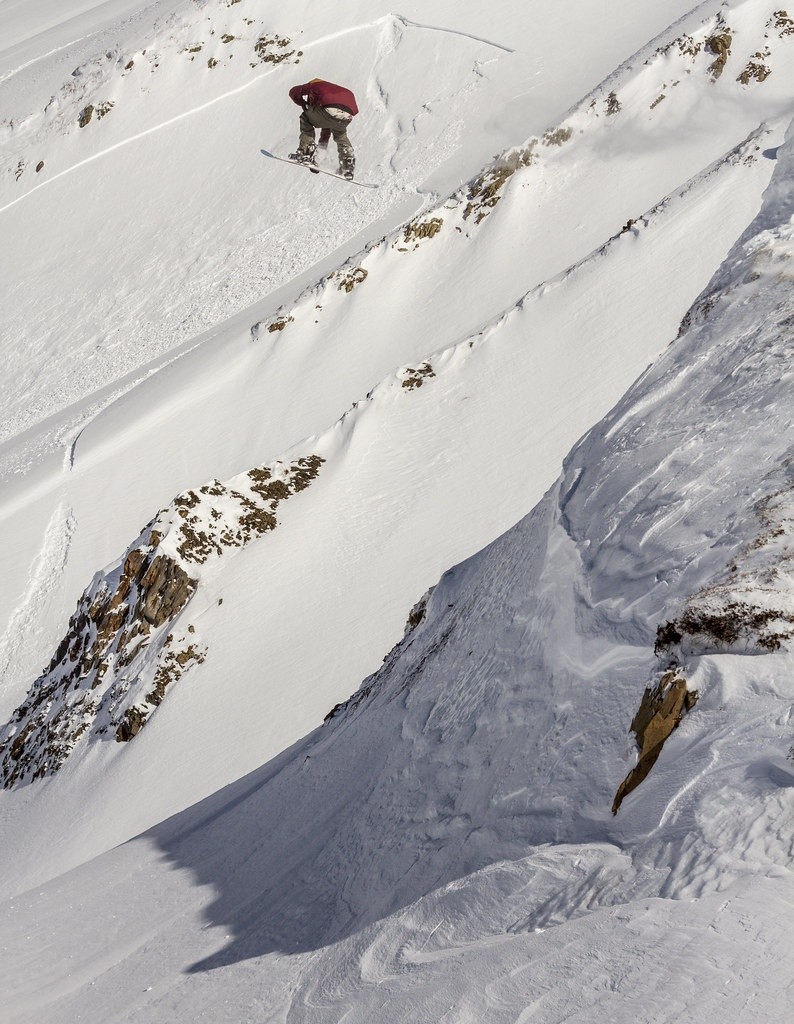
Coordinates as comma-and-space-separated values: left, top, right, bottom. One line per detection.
260, 149, 378, 187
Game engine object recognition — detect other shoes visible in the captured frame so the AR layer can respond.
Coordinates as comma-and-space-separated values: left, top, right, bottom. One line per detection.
288, 154, 317, 165
336, 168, 353, 180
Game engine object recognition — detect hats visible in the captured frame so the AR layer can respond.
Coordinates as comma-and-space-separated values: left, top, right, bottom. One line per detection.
310, 78, 321, 83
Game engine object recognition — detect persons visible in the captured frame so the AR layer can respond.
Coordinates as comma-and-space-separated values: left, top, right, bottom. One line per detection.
289, 77, 358, 179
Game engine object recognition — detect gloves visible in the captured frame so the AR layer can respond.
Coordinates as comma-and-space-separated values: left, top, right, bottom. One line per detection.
318, 139, 328, 149
301, 100, 309, 111
